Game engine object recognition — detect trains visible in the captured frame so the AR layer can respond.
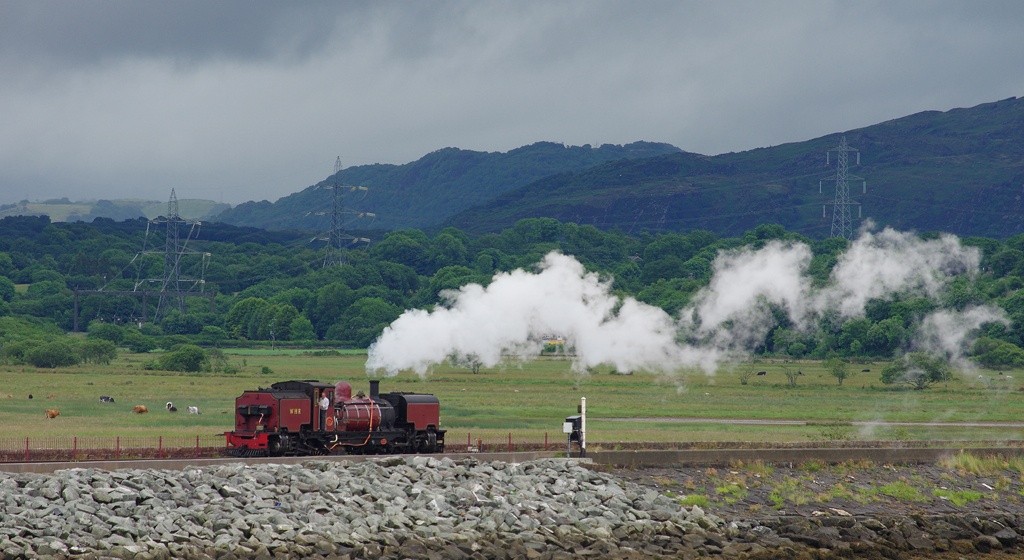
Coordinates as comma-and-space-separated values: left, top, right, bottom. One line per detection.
225, 379, 448, 455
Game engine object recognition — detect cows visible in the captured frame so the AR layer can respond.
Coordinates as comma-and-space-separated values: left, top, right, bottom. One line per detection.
166, 402, 178, 412
99, 395, 115, 403
132, 404, 148, 414
186, 406, 201, 415
45, 408, 61, 419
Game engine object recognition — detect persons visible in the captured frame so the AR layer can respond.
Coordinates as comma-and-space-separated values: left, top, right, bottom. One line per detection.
317, 392, 329, 432
